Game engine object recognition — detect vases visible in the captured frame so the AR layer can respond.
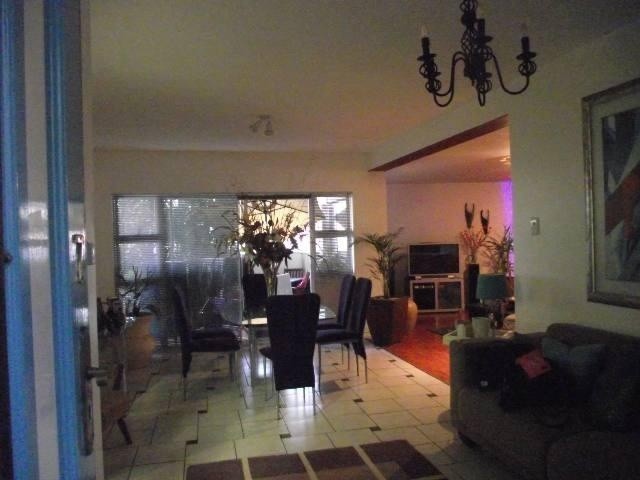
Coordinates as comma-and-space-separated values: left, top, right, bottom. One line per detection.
468, 264, 480, 302
262, 273, 277, 297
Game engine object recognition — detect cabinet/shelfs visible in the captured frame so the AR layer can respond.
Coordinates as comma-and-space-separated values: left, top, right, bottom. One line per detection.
407, 279, 465, 314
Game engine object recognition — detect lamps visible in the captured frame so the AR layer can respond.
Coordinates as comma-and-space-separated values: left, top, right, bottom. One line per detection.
416, 0, 537, 106
477, 274, 515, 329
250, 113, 275, 136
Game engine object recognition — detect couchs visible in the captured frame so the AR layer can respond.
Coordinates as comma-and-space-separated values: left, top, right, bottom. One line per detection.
448, 322, 640, 480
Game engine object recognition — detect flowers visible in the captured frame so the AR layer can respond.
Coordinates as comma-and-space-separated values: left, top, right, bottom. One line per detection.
459, 228, 492, 264
210, 195, 310, 298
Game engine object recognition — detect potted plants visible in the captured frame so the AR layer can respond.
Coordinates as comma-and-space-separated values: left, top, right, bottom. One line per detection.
482, 224, 515, 274
114, 265, 157, 353
347, 225, 419, 347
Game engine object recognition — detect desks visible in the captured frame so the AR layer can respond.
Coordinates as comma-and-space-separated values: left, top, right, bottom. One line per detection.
237, 305, 338, 378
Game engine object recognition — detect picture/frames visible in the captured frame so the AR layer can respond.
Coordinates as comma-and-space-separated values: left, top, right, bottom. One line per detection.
580, 78, 640, 310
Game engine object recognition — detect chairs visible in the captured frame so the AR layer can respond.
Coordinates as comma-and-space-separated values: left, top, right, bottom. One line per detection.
101, 390, 148, 446
282, 268, 305, 287
317, 274, 356, 370
171, 274, 241, 401
312, 277, 371, 390
259, 293, 321, 420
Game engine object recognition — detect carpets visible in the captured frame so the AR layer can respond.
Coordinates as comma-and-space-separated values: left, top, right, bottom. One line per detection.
186, 438, 448, 480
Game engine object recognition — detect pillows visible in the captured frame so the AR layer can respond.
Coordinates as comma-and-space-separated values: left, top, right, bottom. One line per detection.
501, 333, 639, 430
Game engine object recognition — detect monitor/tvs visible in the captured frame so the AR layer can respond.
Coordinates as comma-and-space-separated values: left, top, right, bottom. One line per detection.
408, 241, 461, 277
